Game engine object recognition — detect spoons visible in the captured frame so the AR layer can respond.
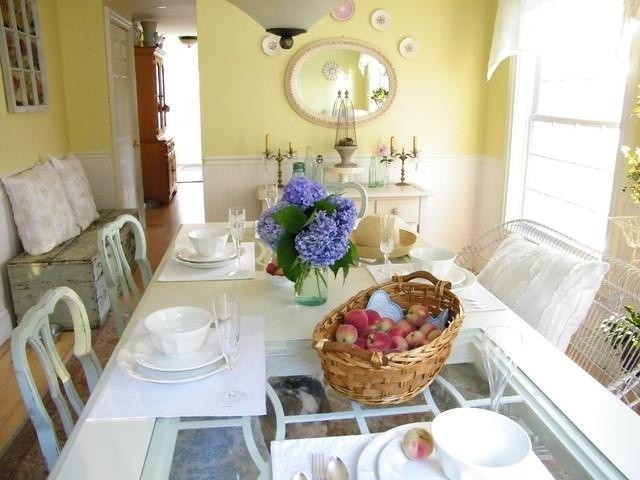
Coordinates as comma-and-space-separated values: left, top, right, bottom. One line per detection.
327, 457, 350, 480
293, 470, 307, 480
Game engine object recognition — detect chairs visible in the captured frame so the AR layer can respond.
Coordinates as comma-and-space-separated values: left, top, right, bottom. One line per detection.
94, 212, 153, 342
322, 180, 370, 222
8, 285, 103, 476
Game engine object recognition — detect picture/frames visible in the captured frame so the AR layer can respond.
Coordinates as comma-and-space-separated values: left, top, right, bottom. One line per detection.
0, 0, 54, 115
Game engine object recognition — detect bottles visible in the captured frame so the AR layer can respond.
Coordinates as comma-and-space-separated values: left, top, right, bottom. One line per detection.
367, 156, 377, 187
292, 144, 324, 187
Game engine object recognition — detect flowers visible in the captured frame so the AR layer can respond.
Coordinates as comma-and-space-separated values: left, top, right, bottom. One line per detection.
256, 173, 359, 296
375, 143, 395, 169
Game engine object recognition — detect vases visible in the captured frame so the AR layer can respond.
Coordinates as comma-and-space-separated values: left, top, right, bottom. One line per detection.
293, 255, 330, 307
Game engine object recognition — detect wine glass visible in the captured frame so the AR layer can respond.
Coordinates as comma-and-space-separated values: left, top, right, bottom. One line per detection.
376, 213, 400, 273
211, 291, 251, 405
228, 207, 253, 278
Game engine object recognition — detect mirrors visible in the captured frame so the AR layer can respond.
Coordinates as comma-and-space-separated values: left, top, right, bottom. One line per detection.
282, 37, 400, 129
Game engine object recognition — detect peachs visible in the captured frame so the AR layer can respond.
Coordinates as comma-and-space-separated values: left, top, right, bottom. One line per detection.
402, 428, 433, 461
335, 304, 441, 353
266, 263, 277, 273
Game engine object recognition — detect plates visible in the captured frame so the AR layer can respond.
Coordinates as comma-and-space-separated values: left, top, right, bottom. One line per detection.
399, 36, 420, 57
117, 330, 228, 385
330, 0, 355, 21
370, 8, 394, 31
356, 421, 556, 480
262, 35, 280, 56
439, 267, 476, 293
173, 245, 245, 271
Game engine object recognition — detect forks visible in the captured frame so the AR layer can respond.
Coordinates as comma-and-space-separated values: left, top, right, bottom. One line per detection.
309, 451, 328, 480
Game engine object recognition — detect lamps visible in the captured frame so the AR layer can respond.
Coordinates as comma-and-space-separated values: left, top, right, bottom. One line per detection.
228, 1, 344, 53
178, 34, 197, 48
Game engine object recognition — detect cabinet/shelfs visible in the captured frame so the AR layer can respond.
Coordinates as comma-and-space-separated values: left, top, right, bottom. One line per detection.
141, 135, 179, 206
254, 182, 427, 236
133, 42, 170, 140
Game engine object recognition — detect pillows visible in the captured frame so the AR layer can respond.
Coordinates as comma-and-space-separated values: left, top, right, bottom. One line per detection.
0, 158, 82, 257
475, 228, 612, 385
43, 147, 101, 232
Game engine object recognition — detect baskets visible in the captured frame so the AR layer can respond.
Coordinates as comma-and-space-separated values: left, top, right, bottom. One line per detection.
311, 271, 465, 406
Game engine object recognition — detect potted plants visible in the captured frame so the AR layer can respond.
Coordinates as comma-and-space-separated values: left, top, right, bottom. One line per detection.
598, 305, 640, 380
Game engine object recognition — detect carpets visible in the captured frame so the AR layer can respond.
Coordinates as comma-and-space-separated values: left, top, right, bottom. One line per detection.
0, 309, 572, 479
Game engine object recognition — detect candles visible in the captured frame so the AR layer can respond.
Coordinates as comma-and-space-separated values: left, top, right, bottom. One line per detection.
390, 135, 397, 150
289, 141, 294, 152
412, 135, 417, 151
265, 132, 272, 151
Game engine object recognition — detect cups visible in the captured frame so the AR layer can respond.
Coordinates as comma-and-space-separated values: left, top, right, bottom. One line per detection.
264, 183, 278, 211
407, 245, 458, 280
431, 406, 531, 480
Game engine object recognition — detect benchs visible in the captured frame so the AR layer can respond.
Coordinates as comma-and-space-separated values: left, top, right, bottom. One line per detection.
454, 214, 639, 413
4, 205, 139, 337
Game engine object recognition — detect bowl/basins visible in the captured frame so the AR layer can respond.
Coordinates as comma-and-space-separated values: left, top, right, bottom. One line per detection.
187, 226, 231, 256
143, 305, 212, 353
350, 229, 417, 259
